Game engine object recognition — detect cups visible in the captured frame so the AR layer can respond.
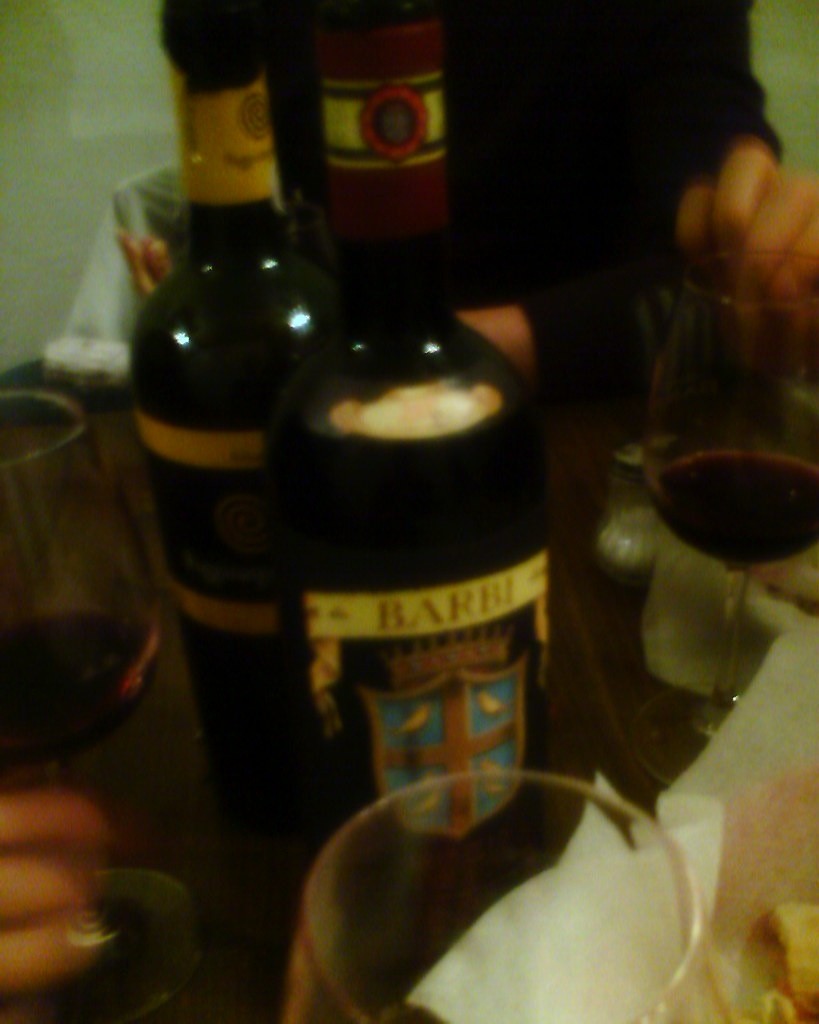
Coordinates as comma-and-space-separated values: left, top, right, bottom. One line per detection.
282, 767, 726, 1023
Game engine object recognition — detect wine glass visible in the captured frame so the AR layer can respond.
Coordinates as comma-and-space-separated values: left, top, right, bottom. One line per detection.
0, 389, 201, 1024
629, 252, 819, 787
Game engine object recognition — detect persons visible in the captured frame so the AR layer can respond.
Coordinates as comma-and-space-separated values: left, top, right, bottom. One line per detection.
158, 1, 817, 391
0, 790, 114, 1024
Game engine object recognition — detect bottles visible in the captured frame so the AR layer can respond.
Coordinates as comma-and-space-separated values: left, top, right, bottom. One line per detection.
595, 442, 658, 588
126, 0, 555, 891
